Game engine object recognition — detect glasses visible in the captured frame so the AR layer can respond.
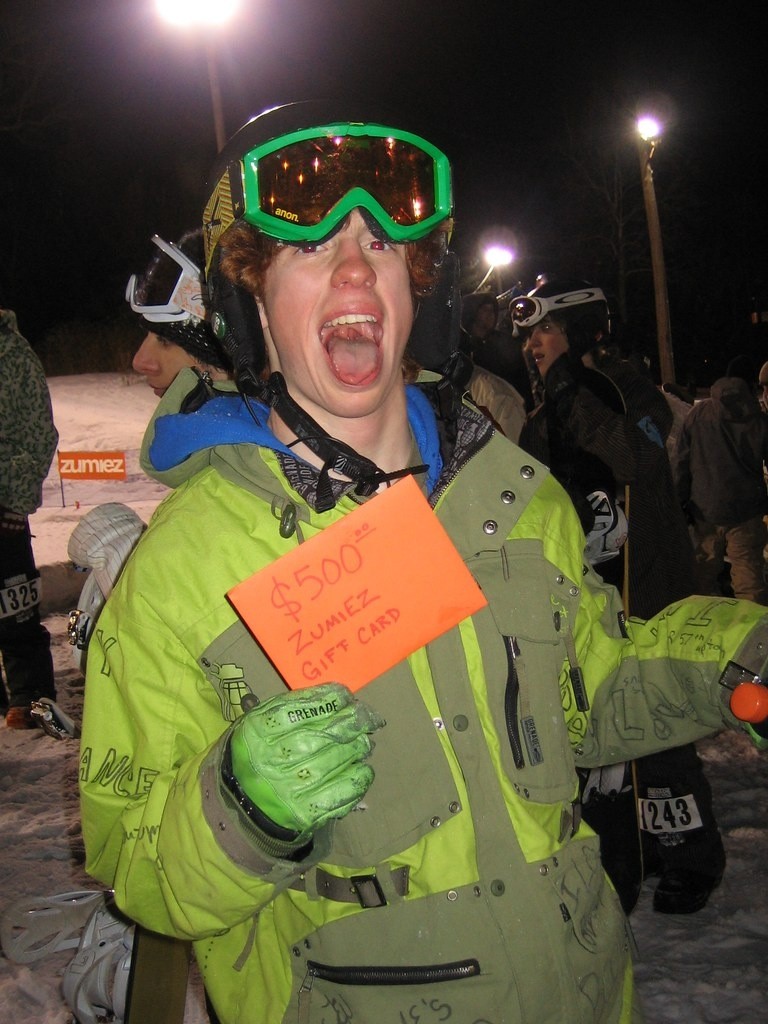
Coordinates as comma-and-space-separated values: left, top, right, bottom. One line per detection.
235, 122, 454, 244
124, 235, 210, 322
510, 293, 562, 336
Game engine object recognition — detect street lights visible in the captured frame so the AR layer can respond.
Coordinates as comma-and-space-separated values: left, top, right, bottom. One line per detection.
634, 117, 679, 391
154, 0, 239, 155
486, 246, 514, 296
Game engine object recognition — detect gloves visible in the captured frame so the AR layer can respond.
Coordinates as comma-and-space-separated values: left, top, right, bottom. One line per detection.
216, 681, 389, 844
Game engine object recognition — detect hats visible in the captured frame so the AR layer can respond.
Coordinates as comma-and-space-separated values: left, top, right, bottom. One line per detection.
726, 356, 758, 383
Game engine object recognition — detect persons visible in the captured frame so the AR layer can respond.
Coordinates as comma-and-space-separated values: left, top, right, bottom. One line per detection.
0, 308, 60, 728
68, 102, 768, 1024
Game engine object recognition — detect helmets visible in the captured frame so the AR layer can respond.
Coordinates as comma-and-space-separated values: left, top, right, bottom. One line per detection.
523, 274, 612, 337
140, 228, 231, 372
208, 102, 460, 368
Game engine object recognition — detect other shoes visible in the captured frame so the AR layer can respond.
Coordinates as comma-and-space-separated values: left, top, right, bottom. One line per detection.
601, 858, 649, 915
7, 701, 37, 731
653, 867, 724, 915
